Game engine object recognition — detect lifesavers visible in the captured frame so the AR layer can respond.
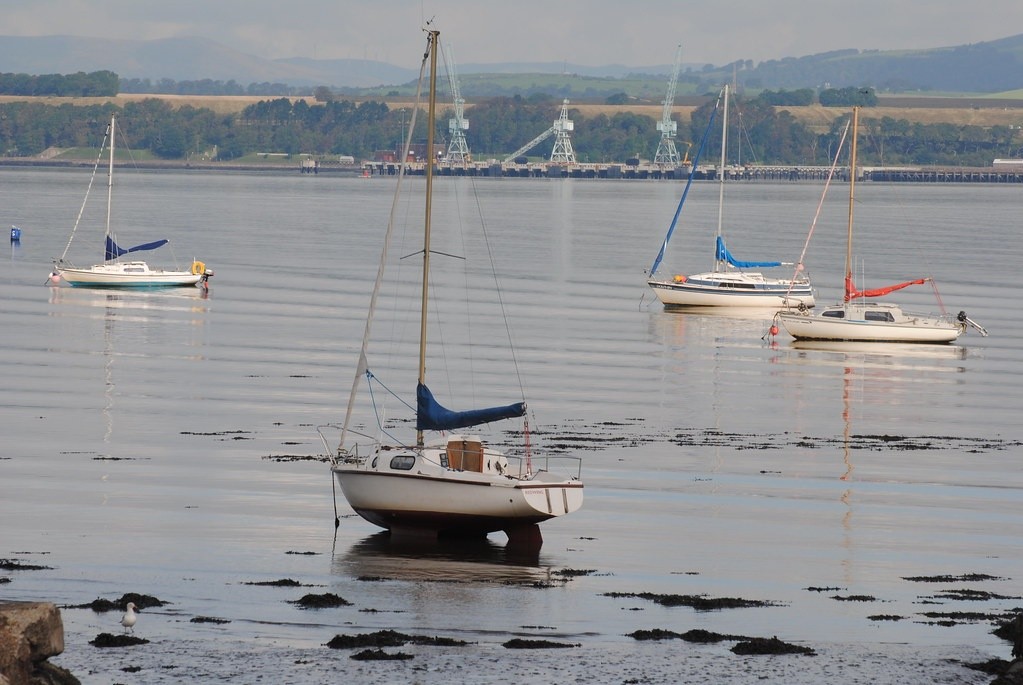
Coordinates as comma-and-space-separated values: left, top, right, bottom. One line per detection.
192, 261, 204, 275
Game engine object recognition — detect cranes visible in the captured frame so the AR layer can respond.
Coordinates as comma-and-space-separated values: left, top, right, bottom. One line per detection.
443, 42, 471, 161
505, 97, 577, 164
654, 43, 694, 167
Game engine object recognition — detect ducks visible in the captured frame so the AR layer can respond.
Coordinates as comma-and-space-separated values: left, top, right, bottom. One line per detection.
117, 602, 138, 634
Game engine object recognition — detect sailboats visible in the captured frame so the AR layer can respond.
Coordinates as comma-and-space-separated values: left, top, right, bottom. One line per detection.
52, 111, 214, 288
771, 103, 990, 344
645, 81, 816, 310
318, 16, 586, 523
